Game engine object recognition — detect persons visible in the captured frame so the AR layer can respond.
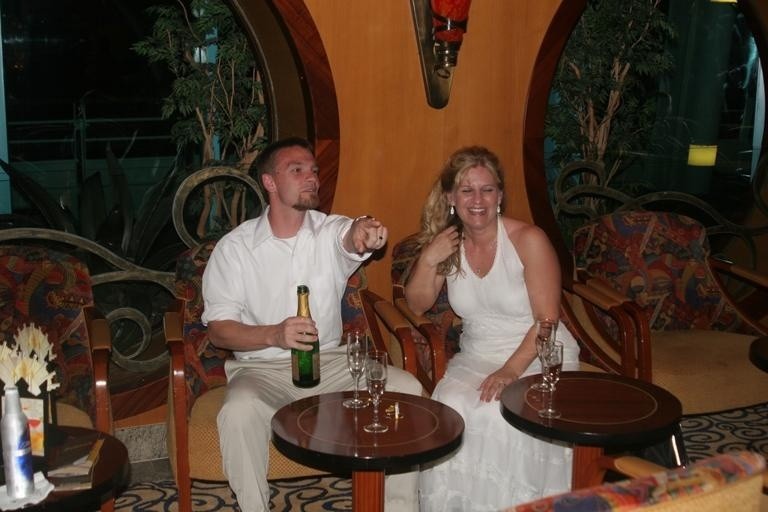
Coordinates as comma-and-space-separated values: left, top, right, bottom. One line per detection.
398, 144, 580, 511
199, 136, 429, 511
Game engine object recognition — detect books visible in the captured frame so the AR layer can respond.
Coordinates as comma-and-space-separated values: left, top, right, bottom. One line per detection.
45, 434, 105, 493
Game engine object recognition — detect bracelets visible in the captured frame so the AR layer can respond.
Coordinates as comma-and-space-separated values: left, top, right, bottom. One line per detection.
351, 214, 375, 223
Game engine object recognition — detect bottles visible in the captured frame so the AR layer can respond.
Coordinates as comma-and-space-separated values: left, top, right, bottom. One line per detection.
289, 284, 320, 390
0, 385, 36, 504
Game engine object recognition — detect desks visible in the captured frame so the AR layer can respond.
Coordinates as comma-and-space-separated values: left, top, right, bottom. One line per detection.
500, 372, 684, 493
270, 391, 465, 511
1, 425, 130, 510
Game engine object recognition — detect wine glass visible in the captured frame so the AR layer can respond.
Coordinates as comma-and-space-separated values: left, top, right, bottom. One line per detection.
342, 332, 369, 409
529, 316, 560, 394
535, 340, 565, 421
362, 350, 390, 434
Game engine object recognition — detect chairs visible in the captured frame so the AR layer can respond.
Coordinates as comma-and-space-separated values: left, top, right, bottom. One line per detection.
393, 233, 638, 490
1, 245, 113, 512
513, 452, 766, 512
572, 211, 766, 460
162, 239, 417, 511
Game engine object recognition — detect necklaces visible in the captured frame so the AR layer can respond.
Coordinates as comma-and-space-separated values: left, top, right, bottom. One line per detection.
465, 250, 497, 277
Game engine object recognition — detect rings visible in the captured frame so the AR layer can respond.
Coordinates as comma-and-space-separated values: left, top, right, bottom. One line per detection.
377, 235, 385, 241
499, 381, 505, 384
